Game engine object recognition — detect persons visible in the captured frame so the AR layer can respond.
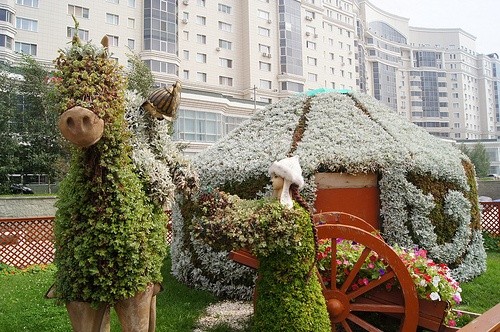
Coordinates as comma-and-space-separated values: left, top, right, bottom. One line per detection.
250, 155, 332, 332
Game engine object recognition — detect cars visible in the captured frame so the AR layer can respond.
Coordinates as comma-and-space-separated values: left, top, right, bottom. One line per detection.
9, 183, 34, 194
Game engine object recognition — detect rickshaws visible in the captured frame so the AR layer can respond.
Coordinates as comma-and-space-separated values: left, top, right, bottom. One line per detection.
227, 212, 500, 332
